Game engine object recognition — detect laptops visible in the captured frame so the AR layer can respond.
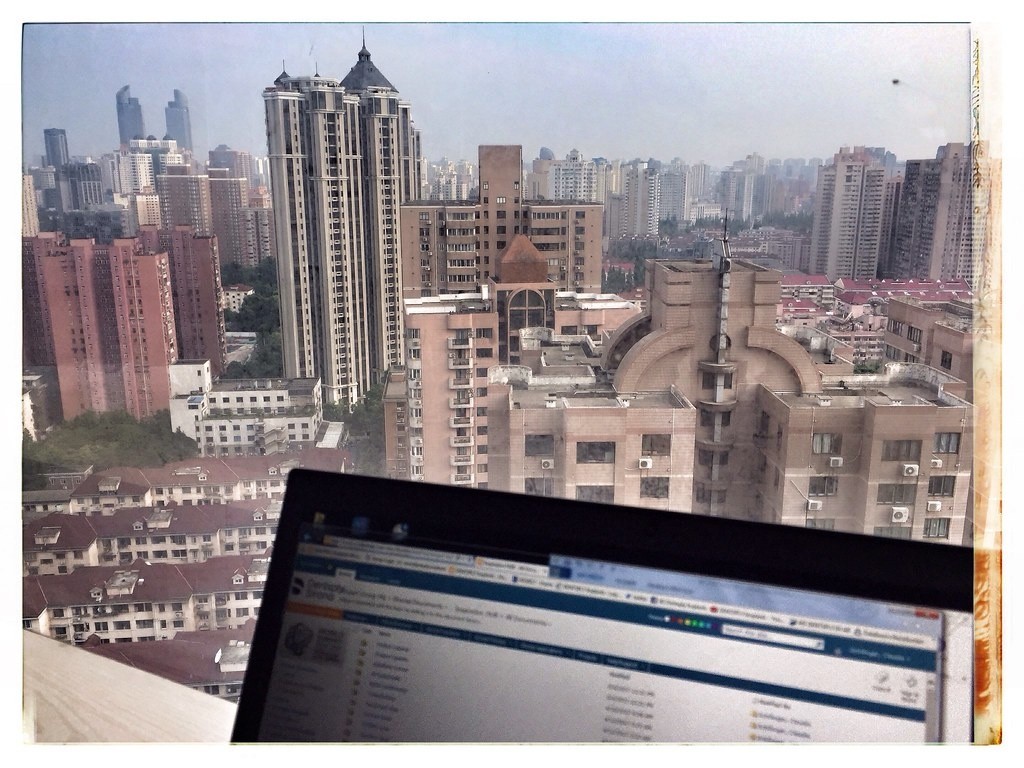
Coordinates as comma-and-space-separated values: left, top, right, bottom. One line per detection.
232, 467, 978, 742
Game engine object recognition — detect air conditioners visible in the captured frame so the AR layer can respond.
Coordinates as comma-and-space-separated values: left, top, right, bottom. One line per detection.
927, 500, 941, 511
418, 476, 424, 481
901, 464, 919, 477
807, 500, 823, 511
638, 458, 652, 469
890, 507, 909, 522
468, 332, 475, 338
931, 460, 942, 468
913, 344, 919, 351
449, 352, 455, 358
541, 458, 554, 469
422, 220, 432, 270
442, 221, 445, 226
175, 613, 182, 618
415, 400, 421, 405
560, 266, 566, 271
416, 381, 421, 385
416, 439, 422, 444
426, 282, 432, 287
829, 456, 843, 468
